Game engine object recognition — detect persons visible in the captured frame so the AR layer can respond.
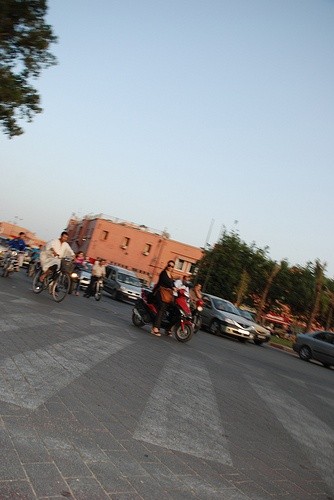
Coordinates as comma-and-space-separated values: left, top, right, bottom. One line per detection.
40, 231, 75, 285
151, 260, 203, 337
267, 322, 297, 343
3, 232, 44, 273
74, 250, 106, 296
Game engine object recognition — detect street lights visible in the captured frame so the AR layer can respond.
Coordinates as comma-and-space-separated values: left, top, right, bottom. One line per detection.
203, 241, 216, 292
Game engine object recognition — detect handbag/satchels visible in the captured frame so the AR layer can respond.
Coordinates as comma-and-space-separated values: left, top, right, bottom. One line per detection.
159, 286, 174, 303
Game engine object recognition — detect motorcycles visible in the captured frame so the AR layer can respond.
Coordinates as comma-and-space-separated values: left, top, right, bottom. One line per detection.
0, 248, 25, 277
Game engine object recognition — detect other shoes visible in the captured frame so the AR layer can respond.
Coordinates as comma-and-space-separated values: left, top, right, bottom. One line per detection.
15, 268, 20, 272
72, 290, 80, 296
83, 294, 90, 298
37, 275, 44, 287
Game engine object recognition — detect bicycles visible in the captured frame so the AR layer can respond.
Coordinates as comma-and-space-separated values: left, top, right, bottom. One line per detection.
32, 254, 75, 303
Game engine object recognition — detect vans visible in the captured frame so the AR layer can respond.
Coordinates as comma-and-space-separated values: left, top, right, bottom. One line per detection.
102, 264, 143, 303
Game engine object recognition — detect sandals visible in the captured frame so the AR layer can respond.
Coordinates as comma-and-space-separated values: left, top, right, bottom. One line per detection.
151, 328, 161, 336
164, 332, 173, 336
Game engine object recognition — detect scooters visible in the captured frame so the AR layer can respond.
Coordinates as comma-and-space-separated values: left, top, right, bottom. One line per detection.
65, 266, 85, 294
132, 287, 194, 343
88, 273, 106, 301
189, 299, 203, 334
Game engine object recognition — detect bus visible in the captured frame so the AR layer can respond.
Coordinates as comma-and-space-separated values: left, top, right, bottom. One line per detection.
244, 308, 292, 340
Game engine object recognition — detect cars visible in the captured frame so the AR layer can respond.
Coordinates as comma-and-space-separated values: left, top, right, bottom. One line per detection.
200, 293, 258, 343
79, 262, 93, 291
0, 236, 23, 267
238, 310, 271, 345
23, 247, 31, 268
293, 331, 334, 367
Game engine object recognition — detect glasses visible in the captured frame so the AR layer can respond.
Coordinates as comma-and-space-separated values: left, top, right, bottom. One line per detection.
168, 264, 174, 268
